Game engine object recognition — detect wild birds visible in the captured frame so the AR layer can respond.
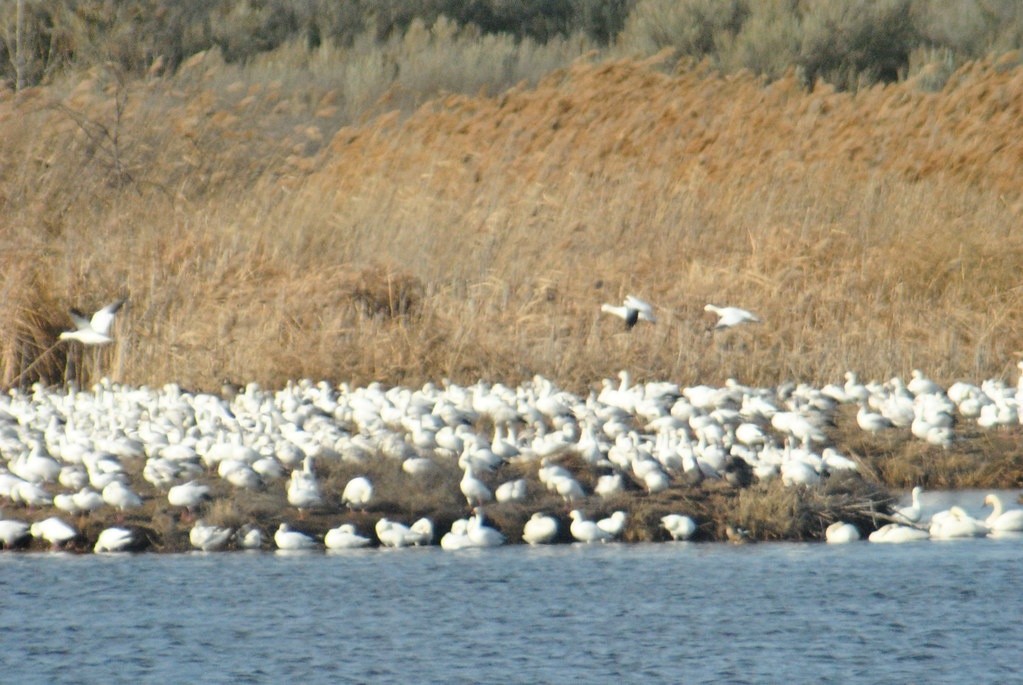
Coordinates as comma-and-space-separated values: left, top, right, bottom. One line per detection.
0, 360, 1023, 553
56, 299, 126, 345
600, 296, 657, 330
703, 304, 763, 330
